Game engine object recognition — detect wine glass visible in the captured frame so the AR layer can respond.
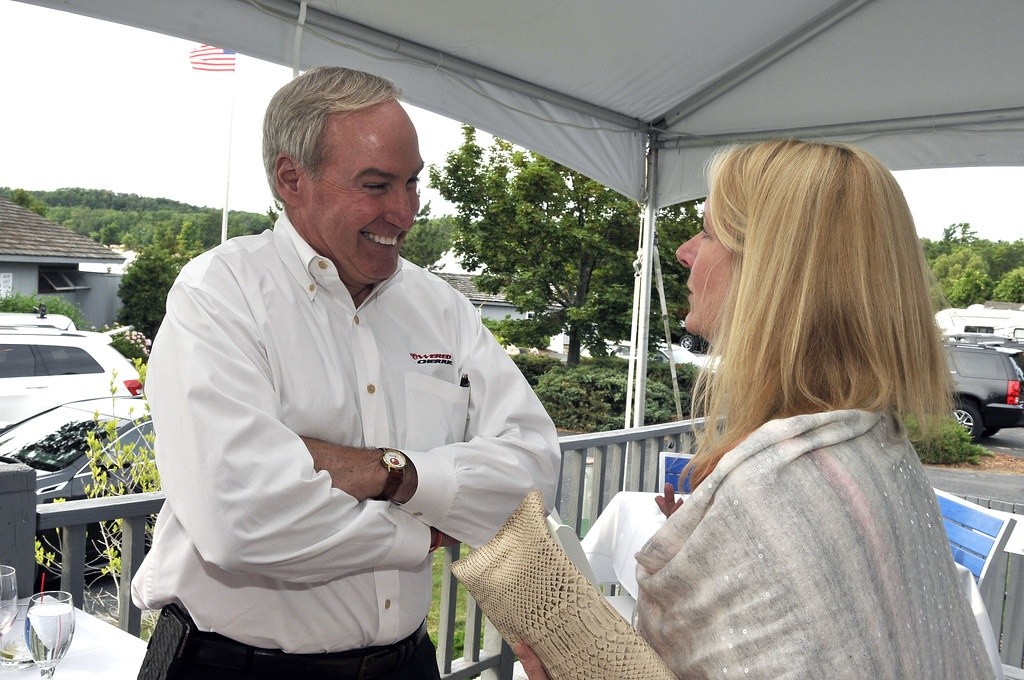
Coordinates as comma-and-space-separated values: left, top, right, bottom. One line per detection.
0, 565, 17, 649
25, 591, 75, 680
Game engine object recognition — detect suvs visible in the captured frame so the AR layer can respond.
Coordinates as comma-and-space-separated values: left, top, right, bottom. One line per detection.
931, 307, 1024, 444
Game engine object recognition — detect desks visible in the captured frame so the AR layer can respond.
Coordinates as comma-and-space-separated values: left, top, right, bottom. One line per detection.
0, 593, 149, 680
580, 492, 1005, 680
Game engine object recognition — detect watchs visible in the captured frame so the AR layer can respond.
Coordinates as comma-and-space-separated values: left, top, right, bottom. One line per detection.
370, 445, 407, 499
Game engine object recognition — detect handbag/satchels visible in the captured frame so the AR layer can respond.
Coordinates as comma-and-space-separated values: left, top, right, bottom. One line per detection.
449, 490, 678, 680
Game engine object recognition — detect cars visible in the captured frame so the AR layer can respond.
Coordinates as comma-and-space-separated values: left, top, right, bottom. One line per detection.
581, 338, 703, 372
0, 323, 148, 439
0, 396, 159, 584
0, 309, 78, 332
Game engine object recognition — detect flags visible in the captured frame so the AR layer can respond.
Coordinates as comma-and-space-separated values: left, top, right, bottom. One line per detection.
190, 45, 237, 71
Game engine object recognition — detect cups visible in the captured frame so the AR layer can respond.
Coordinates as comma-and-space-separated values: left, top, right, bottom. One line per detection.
0, 604, 36, 671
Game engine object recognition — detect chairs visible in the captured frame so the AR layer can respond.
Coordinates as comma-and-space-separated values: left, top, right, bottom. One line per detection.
547, 505, 636, 623
659, 451, 695, 495
933, 487, 1017, 610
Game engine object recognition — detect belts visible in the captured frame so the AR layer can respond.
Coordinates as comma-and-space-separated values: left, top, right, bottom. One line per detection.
181, 616, 426, 680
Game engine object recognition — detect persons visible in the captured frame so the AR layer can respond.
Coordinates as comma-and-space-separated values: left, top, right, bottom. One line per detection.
130, 66, 563, 680
512, 136, 998, 680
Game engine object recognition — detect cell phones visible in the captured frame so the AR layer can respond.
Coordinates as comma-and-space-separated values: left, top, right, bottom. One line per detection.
137, 604, 191, 680
